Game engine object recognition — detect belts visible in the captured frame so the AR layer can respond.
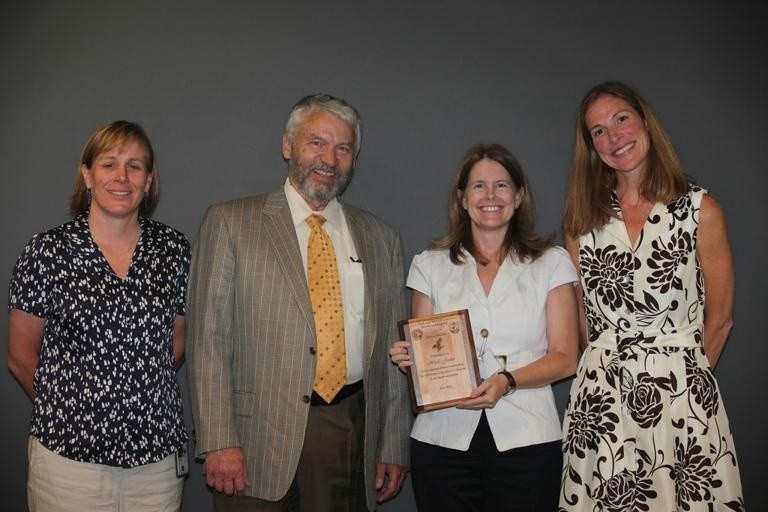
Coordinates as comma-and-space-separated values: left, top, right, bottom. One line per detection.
310, 377, 364, 406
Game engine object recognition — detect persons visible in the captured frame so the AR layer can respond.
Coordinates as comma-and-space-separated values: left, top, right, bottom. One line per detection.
553, 80, 748, 511
180, 93, 412, 512
388, 143, 580, 512
8, 119, 191, 512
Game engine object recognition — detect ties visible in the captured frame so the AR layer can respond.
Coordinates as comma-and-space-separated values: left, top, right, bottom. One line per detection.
305, 214, 348, 404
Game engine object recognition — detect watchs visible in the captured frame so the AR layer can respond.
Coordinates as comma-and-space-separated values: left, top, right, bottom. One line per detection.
494, 371, 515, 398
